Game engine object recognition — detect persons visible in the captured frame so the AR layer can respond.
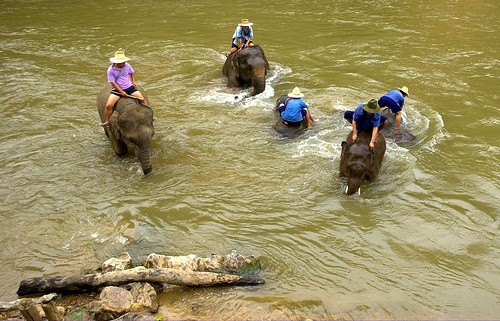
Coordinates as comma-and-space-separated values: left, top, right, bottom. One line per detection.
278, 87, 316, 129
98, 51, 157, 126
225, 19, 254, 57
344, 99, 389, 150
378, 86, 410, 130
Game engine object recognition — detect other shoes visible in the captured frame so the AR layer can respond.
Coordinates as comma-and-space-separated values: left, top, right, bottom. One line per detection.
98, 123, 110, 127
152, 117, 157, 121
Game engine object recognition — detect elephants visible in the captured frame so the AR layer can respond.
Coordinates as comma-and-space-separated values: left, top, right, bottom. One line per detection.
338, 129, 386, 196
97, 81, 157, 175
272, 94, 309, 139
223, 44, 270, 104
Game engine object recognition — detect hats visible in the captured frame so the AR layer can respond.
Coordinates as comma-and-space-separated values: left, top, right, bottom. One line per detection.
287, 88, 305, 98
110, 50, 131, 64
363, 98, 380, 113
398, 86, 409, 96
239, 19, 252, 26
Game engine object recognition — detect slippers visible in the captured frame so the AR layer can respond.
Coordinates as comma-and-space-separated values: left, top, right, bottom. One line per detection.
282, 120, 289, 125
